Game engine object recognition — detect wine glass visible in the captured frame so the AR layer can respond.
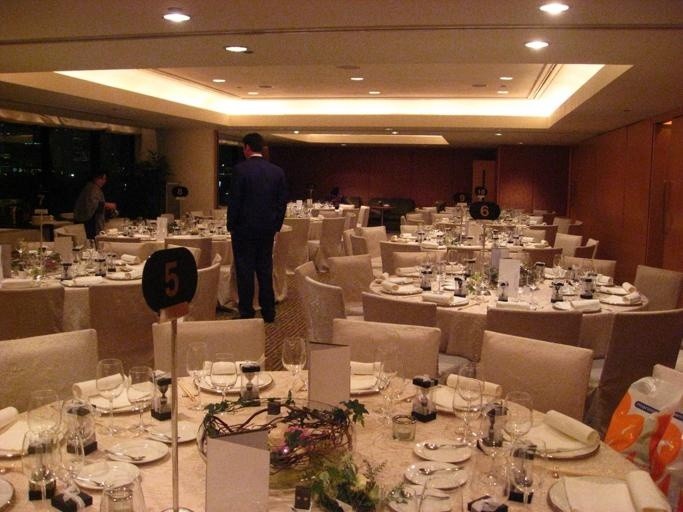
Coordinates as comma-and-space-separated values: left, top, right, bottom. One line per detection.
96, 359, 127, 433
281, 336, 307, 393
501, 391, 534, 456
508, 447, 546, 510
416, 223, 597, 302
455, 362, 485, 437
478, 405, 516, 486
60, 400, 95, 465
372, 345, 402, 415
22, 430, 61, 512
210, 352, 238, 402
454, 380, 481, 443
378, 360, 405, 431
125, 366, 153, 432
11, 222, 217, 283
127, 372, 155, 439
186, 341, 214, 410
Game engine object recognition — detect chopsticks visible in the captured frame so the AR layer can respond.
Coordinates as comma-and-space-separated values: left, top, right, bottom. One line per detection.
179, 380, 197, 402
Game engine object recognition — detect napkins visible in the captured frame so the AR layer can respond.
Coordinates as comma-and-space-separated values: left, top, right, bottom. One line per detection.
425, 372, 500, 413
348, 359, 386, 393
564, 470, 667, 511
71, 373, 151, 409
518, 408, 597, 455
1, 403, 51, 454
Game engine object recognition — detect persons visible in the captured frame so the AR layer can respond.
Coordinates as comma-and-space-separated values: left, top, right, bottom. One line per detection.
227, 133, 288, 324
73, 170, 116, 239
327, 187, 351, 209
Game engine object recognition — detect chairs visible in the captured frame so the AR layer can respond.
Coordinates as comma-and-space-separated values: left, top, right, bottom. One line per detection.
585, 308, 682, 440
2, 193, 682, 384
0, 328, 99, 412
476, 329, 595, 426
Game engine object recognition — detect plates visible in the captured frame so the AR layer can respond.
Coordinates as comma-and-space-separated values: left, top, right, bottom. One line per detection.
143, 419, 200, 445
105, 438, 169, 464
405, 461, 468, 488
515, 436, 602, 460
415, 438, 472, 462
0, 475, 14, 510
193, 370, 274, 393
550, 475, 630, 512
73, 459, 140, 491
388, 485, 453, 511
87, 386, 155, 414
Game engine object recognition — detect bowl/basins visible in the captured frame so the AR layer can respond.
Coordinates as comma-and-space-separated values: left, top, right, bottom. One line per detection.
195, 395, 358, 497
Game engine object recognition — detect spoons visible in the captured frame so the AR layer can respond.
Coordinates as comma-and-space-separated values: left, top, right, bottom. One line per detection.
424, 442, 469, 450
417, 465, 464, 475
398, 490, 450, 500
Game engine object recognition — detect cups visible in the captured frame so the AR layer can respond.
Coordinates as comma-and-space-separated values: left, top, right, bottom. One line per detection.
467, 454, 510, 510
98, 466, 147, 511
418, 472, 465, 511
27, 389, 60, 434
45, 428, 85, 488
392, 413, 416, 443
